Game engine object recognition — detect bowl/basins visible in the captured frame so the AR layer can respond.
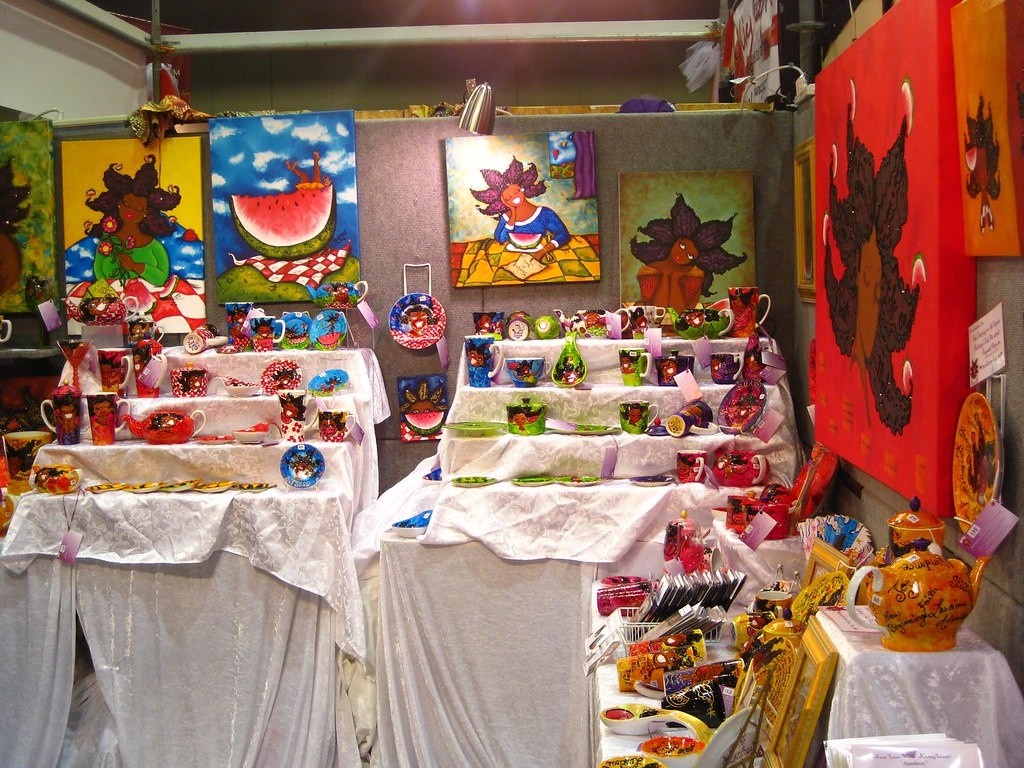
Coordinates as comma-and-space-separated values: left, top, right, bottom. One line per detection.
505, 398, 546, 435
654, 349, 694, 386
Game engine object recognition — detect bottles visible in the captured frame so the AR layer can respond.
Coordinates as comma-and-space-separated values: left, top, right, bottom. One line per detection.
886, 495, 946, 560
742, 334, 765, 382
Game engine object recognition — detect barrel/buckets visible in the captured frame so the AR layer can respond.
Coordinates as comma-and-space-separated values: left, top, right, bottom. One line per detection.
3, 430, 53, 495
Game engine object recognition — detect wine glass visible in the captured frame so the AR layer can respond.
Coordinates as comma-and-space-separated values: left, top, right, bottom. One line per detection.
56, 338, 92, 389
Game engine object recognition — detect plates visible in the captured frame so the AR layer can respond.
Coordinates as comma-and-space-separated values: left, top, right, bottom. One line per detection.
953, 392, 1002, 532
280, 310, 348, 352
792, 450, 838, 519
388, 292, 447, 350
442, 421, 676, 487
85, 432, 326, 495
261, 361, 349, 397
792, 571, 849, 623
717, 380, 767, 435
691, 705, 766, 768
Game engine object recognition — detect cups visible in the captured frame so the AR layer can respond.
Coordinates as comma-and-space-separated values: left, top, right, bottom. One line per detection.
726, 481, 797, 541
464, 311, 552, 388
277, 389, 356, 443
599, 755, 666, 768
677, 449, 709, 483
38, 302, 286, 447
641, 736, 700, 768
663, 512, 703, 574
618, 284, 771, 437
616, 590, 792, 725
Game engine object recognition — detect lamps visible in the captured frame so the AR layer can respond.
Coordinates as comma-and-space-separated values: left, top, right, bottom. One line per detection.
458, 81, 513, 135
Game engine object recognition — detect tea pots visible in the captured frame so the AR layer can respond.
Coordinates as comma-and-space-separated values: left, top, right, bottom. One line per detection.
62, 296, 138, 327
120, 409, 206, 444
846, 551, 992, 651
712, 450, 771, 487
306, 279, 368, 308
553, 308, 632, 340
32, 464, 83, 495
666, 306, 735, 340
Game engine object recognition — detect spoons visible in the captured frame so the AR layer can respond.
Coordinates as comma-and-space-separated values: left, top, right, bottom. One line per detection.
206, 376, 259, 397
600, 703, 713, 743
551, 330, 587, 388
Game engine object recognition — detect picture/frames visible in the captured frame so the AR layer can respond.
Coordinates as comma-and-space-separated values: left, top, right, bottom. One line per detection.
766, 615, 838, 768
793, 135, 815, 303
805, 538, 851, 588
